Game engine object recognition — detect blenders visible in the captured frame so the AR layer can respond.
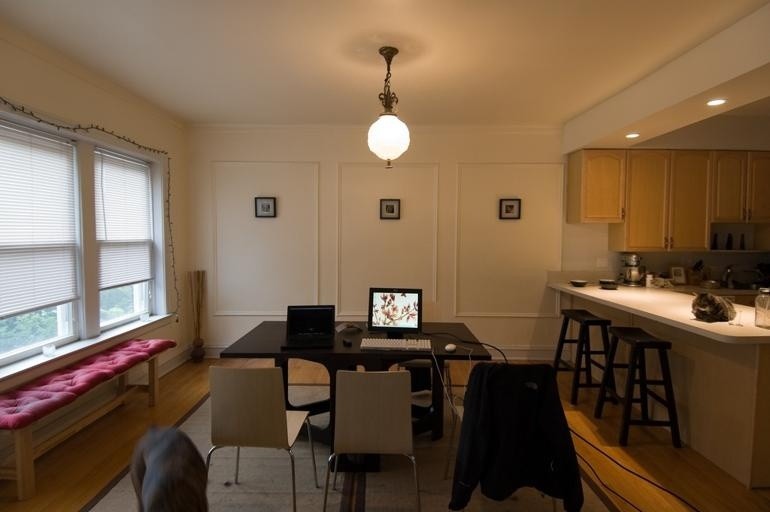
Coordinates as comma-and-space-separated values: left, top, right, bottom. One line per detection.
618, 253, 646, 286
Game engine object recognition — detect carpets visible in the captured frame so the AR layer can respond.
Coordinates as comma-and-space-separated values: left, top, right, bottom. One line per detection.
77, 381, 621, 512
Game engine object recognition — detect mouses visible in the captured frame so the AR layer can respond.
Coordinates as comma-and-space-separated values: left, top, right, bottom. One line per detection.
444, 342, 458, 352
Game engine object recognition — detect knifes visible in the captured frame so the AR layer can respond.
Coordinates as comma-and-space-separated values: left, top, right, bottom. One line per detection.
692, 259, 704, 272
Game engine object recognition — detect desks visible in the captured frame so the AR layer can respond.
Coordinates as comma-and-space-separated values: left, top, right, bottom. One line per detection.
222, 320, 490, 441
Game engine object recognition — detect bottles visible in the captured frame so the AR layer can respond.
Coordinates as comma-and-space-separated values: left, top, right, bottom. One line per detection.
755, 288, 770, 330
646, 275, 653, 288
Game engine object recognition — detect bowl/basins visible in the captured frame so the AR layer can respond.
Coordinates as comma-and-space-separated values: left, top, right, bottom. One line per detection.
570, 280, 588, 287
600, 280, 619, 289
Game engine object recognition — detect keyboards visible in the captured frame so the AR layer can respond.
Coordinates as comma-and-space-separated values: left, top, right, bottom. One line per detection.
358, 337, 432, 352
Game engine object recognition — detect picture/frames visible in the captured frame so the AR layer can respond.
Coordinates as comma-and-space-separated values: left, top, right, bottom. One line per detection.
500, 199, 521, 219
379, 199, 399, 219
255, 197, 277, 217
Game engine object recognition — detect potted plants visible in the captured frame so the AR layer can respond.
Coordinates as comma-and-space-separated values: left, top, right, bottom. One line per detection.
186, 269, 207, 363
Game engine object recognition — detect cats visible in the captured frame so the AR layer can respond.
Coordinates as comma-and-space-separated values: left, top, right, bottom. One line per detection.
691, 291, 736, 322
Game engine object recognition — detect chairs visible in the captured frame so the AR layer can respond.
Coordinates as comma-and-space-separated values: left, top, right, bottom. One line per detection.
448, 363, 584, 512
323, 369, 421, 512
203, 366, 322, 512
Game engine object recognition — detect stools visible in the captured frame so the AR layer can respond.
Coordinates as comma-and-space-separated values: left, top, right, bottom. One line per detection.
551, 309, 619, 405
594, 326, 687, 448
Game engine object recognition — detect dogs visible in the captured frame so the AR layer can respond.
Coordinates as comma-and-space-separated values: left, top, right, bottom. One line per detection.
129, 428, 209, 511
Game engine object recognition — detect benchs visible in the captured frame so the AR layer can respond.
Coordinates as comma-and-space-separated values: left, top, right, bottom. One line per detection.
1, 338, 178, 501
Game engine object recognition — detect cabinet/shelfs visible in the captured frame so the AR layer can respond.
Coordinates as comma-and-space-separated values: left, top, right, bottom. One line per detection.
566, 149, 769, 254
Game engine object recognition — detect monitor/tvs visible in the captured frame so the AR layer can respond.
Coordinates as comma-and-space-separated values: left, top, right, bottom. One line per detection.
367, 285, 423, 335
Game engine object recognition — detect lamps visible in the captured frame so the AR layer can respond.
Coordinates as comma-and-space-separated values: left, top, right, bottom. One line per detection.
367, 46, 413, 169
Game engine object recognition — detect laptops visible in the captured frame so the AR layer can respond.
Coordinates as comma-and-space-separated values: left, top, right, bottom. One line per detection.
280, 304, 336, 351
